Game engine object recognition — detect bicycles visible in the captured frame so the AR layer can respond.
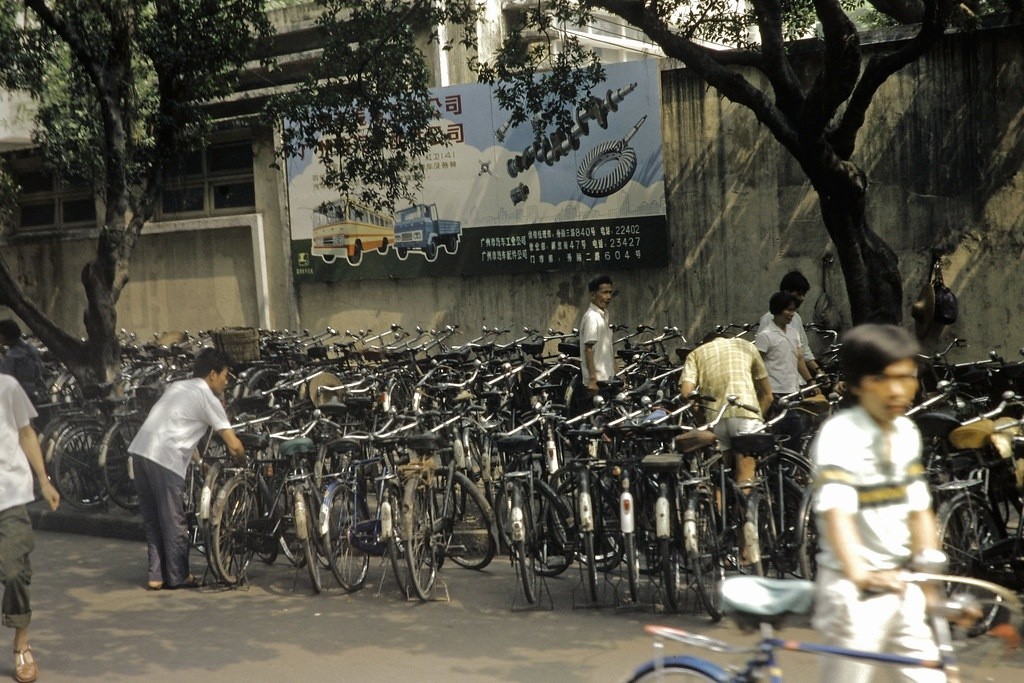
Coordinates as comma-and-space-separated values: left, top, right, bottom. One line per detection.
628, 546, 1024, 683
23, 323, 1024, 612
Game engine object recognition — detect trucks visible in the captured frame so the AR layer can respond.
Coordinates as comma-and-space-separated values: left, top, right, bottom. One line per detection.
394, 201, 462, 261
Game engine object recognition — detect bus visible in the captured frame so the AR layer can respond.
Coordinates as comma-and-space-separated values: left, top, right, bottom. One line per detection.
310, 197, 394, 266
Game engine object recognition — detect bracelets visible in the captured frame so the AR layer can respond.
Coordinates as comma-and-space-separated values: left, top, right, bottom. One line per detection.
807, 378, 815, 384
814, 367, 820, 372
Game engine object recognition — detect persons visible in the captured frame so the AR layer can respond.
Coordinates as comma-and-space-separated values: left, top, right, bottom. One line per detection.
814, 323, 947, 683
127, 347, 247, 589
679, 332, 774, 515
757, 270, 824, 451
1, 319, 54, 429
0, 374, 60, 683
580, 274, 625, 424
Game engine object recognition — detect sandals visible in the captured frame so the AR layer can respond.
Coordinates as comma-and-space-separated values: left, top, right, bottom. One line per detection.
12, 645, 35, 681
146, 572, 205, 590
